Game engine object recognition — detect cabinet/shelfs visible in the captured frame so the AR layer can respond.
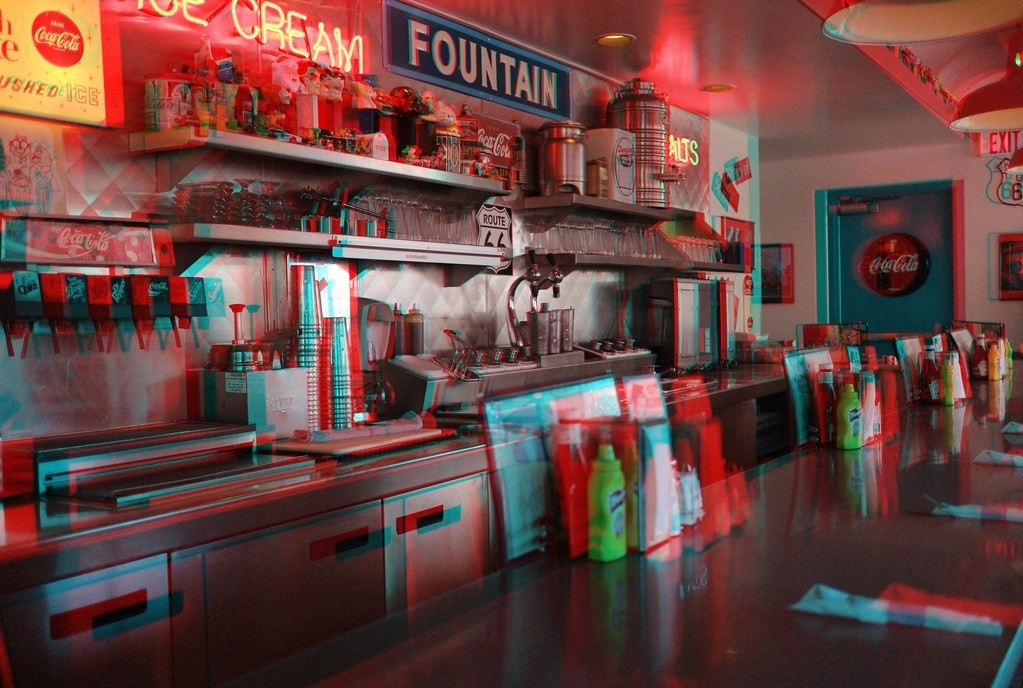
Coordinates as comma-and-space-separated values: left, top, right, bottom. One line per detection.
0, 554, 204, 688
204, 472, 489, 688
525, 194, 745, 272
145, 128, 512, 270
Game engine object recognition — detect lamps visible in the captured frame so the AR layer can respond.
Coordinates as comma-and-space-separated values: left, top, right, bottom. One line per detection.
948, 70, 1022, 132
595, 33, 636, 48
1005, 147, 1023, 175
821, 0, 1023, 45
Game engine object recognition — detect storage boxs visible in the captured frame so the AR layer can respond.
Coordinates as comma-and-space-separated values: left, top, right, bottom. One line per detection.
586, 127, 636, 203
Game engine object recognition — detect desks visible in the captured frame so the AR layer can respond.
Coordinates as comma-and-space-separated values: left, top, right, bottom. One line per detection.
314, 356, 1022, 687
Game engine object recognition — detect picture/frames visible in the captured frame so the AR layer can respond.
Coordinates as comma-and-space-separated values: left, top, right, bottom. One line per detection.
720, 216, 755, 271
751, 243, 782, 303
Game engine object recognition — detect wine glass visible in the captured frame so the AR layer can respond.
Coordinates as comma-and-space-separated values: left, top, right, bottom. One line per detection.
229, 179, 284, 229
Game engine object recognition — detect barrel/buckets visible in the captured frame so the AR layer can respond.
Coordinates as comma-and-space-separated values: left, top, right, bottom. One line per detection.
606, 81, 671, 208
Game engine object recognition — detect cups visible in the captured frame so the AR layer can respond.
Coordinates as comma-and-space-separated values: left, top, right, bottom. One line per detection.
319, 317, 352, 432
560, 310, 575, 351
290, 265, 323, 430
532, 218, 662, 260
349, 192, 479, 246
676, 235, 722, 262
548, 310, 560, 354
527, 310, 549, 356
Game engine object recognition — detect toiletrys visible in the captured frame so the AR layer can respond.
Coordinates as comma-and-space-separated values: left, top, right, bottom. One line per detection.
940, 359, 955, 406
587, 424, 628, 562
835, 371, 861, 450
988, 344, 1000, 380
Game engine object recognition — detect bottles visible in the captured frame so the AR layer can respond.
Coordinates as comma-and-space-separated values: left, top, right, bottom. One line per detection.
974, 333, 989, 378
1007, 341, 1013, 369
391, 303, 405, 358
836, 383, 865, 450
998, 338, 1007, 377
876, 240, 891, 288
942, 360, 955, 406
554, 409, 586, 529
921, 345, 938, 404
586, 444, 627, 561
989, 344, 1001, 381
814, 363, 836, 445
194, 37, 219, 81
404, 304, 424, 355
